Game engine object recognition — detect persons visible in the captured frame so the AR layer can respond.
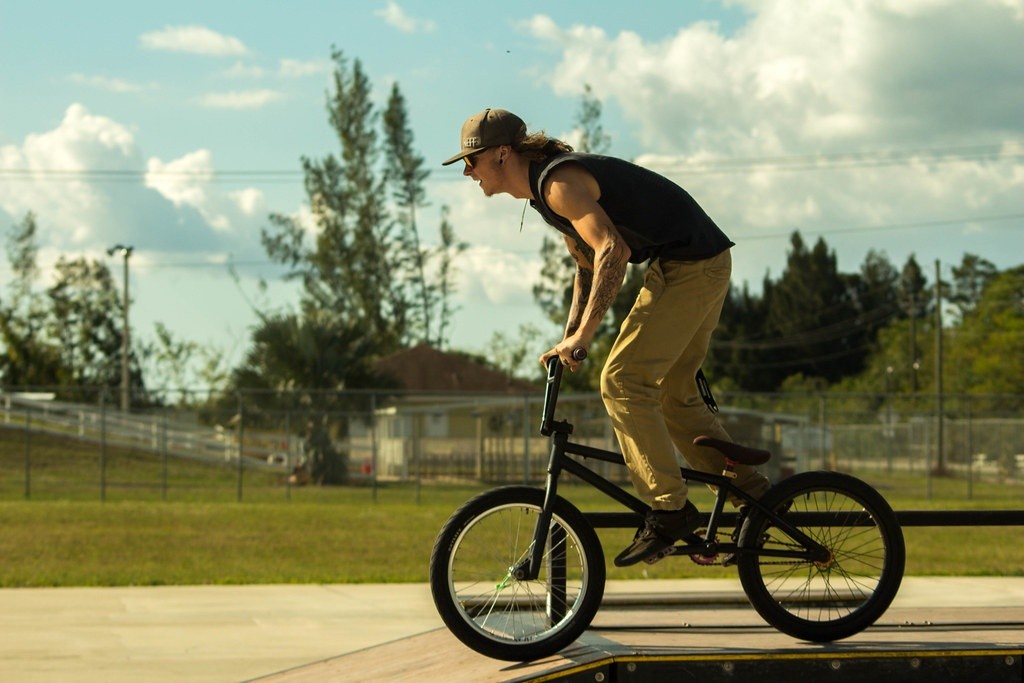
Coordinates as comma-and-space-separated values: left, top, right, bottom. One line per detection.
440, 107, 792, 568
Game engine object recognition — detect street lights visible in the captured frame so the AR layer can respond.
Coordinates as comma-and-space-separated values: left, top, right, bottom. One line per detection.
107, 242, 134, 413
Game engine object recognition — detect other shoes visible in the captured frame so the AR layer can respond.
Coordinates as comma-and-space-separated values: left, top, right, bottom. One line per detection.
613, 500, 701, 567
724, 486, 793, 567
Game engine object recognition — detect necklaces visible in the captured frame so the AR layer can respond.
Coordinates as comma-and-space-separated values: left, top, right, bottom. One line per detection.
519, 196, 529, 232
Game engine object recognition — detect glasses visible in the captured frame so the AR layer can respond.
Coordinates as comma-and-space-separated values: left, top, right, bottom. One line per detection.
462, 145, 501, 169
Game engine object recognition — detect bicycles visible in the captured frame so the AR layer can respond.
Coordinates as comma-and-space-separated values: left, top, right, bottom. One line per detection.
428, 347, 906, 661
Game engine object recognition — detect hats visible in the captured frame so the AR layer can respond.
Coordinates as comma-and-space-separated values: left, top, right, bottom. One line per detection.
442, 107, 526, 166
